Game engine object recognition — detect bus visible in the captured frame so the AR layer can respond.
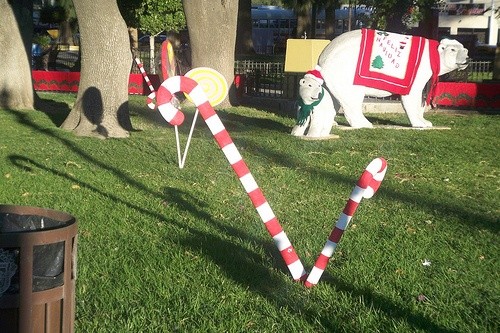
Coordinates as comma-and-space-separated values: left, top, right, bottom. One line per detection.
248, 6, 376, 57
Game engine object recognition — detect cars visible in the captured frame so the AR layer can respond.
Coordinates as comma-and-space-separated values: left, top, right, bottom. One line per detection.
139, 34, 166, 44
31, 44, 79, 71
439, 33, 497, 68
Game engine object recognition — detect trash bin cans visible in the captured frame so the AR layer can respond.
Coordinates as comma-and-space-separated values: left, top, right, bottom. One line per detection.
0, 203, 79, 332
